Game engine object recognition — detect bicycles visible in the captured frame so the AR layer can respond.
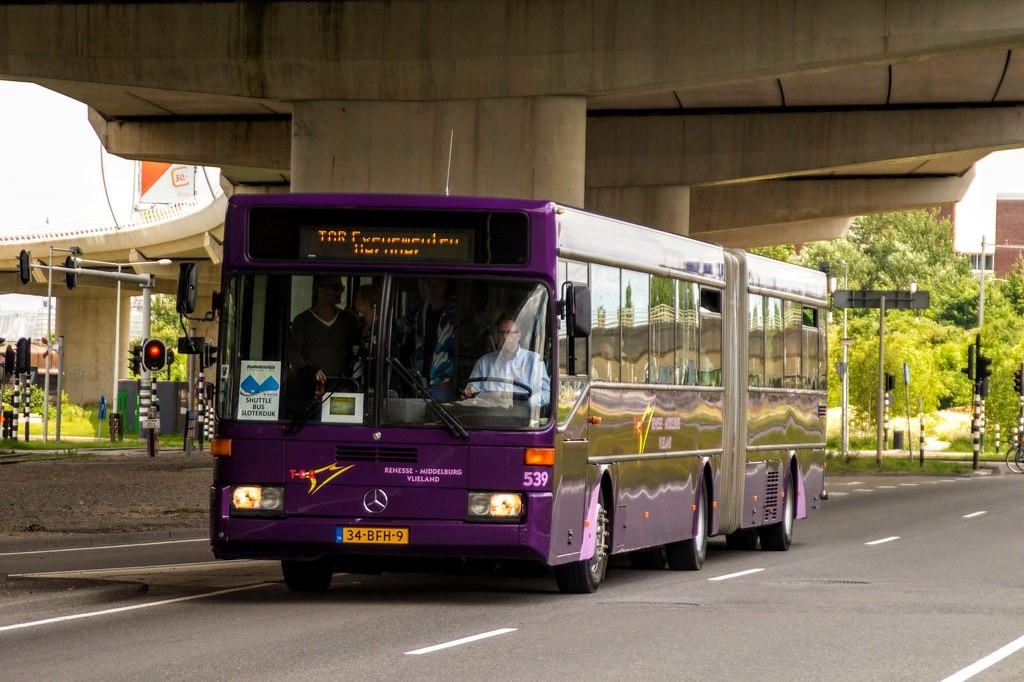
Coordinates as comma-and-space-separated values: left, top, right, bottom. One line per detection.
1005, 431, 1024, 474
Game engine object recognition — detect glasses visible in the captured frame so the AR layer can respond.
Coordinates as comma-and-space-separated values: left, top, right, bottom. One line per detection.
498, 330, 518, 336
320, 283, 346, 292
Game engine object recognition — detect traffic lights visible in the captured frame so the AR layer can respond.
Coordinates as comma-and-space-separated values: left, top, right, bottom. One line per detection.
143, 338, 167, 372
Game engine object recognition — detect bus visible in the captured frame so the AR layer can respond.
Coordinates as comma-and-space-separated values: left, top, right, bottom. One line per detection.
208, 192, 830, 595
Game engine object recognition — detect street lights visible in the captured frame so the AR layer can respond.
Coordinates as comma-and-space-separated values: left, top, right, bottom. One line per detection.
43, 245, 84, 445
69, 256, 173, 438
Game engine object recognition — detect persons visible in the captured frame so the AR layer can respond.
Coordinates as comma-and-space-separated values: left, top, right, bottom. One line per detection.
282, 271, 551, 419
599, 334, 628, 382
